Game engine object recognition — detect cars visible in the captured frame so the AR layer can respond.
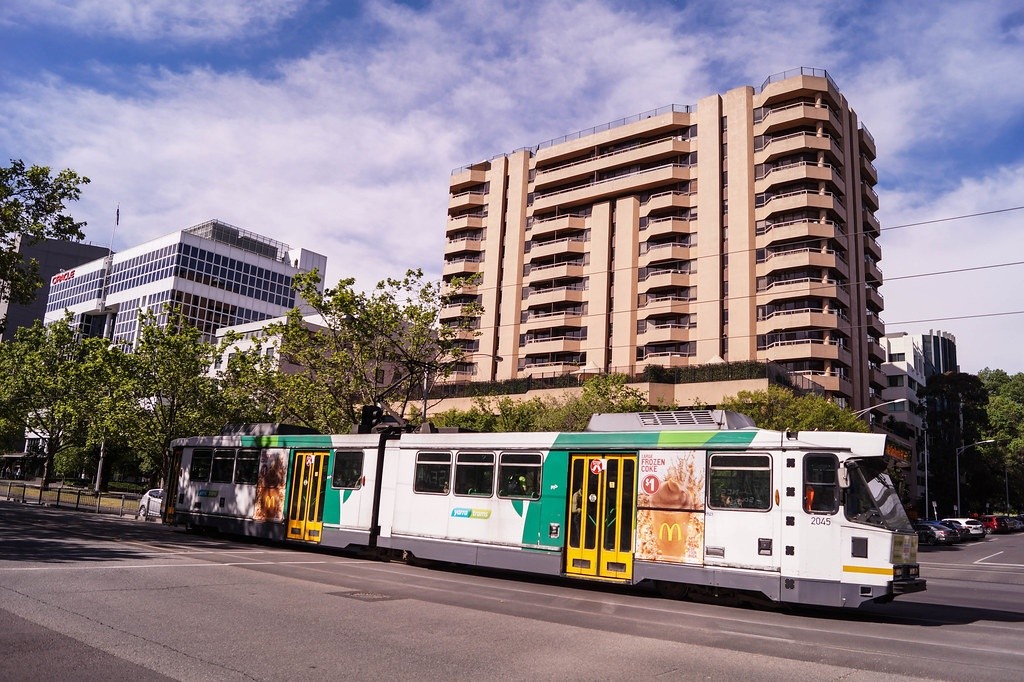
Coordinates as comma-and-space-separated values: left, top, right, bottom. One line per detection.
909, 514, 1024, 545
138, 488, 164, 517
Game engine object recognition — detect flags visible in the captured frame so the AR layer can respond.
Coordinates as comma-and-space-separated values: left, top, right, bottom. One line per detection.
117, 208, 119, 225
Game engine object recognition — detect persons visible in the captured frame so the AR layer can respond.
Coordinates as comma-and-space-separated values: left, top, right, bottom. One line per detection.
1, 465, 21, 480
572, 482, 592, 547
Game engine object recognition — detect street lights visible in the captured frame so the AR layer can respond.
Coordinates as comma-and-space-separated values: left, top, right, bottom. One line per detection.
850, 399, 908, 419
956, 440, 995, 518
345, 313, 504, 423
1006, 466, 1010, 513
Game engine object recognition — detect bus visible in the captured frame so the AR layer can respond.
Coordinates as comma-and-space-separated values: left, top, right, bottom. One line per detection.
164, 410, 927, 619
